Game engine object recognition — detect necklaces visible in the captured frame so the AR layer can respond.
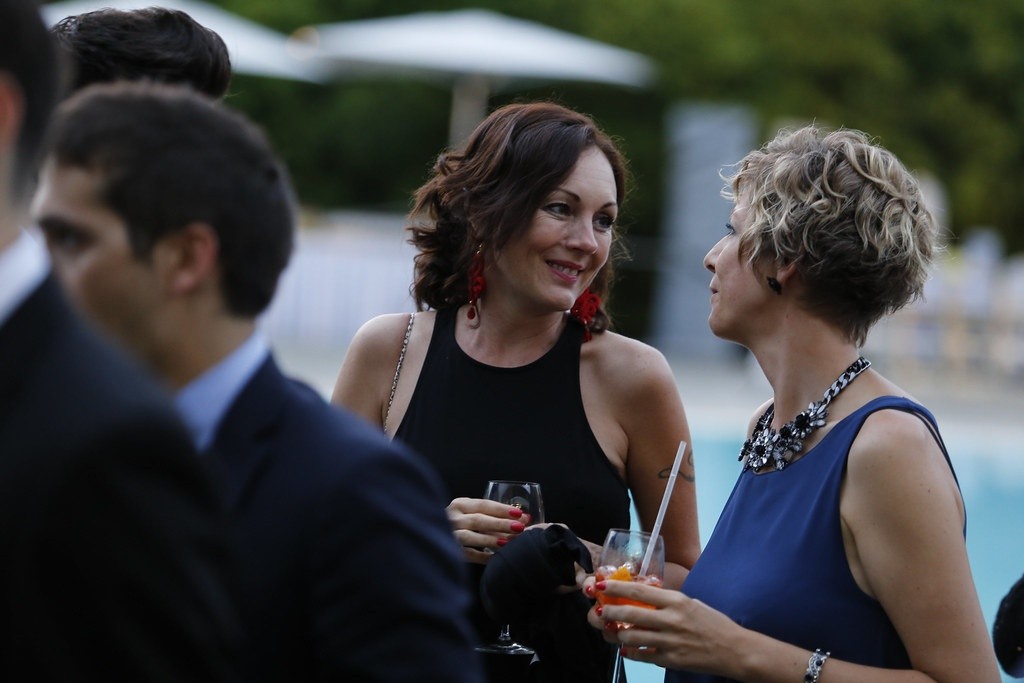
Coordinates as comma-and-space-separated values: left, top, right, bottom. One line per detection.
738, 358, 868, 471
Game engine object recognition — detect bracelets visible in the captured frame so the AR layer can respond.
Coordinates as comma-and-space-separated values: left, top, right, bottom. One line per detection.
804, 649, 829, 683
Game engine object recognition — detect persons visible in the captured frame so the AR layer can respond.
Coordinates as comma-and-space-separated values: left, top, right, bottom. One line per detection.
584, 128, 1004, 683
331, 101, 702, 683
29, 85, 479, 683
0, 0, 233, 683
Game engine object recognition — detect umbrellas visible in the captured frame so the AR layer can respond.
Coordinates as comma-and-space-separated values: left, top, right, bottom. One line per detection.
40, 0, 291, 79
300, 5, 640, 144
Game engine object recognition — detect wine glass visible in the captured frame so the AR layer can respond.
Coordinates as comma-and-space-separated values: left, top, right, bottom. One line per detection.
474, 480, 544, 655
596, 528, 665, 683
